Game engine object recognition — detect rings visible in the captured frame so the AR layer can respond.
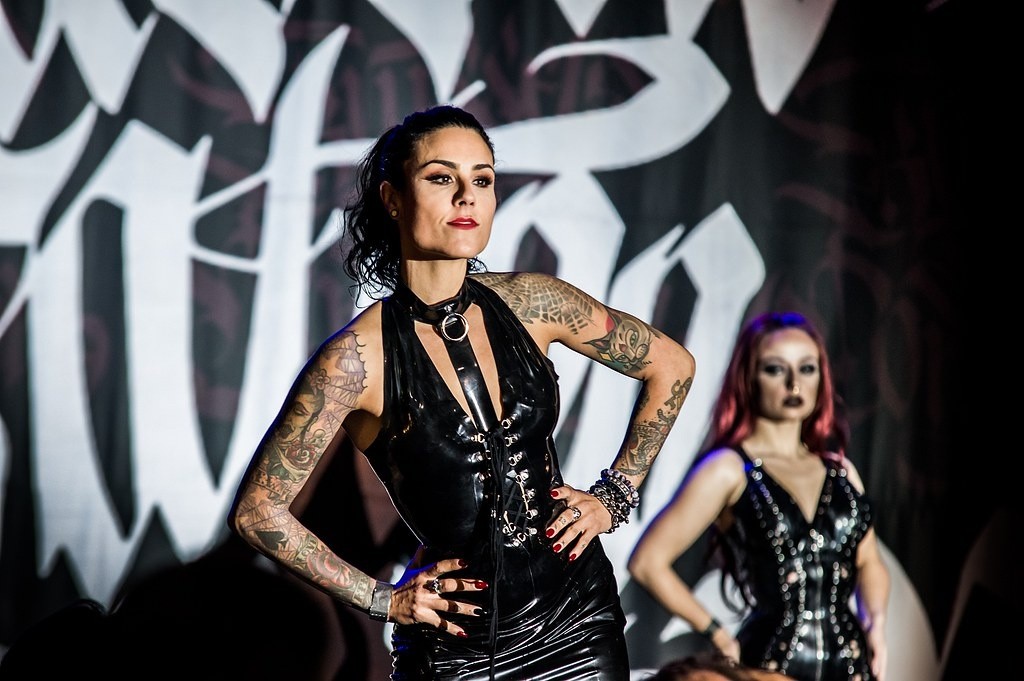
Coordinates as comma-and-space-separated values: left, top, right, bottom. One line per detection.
428, 578, 442, 594
568, 506, 582, 522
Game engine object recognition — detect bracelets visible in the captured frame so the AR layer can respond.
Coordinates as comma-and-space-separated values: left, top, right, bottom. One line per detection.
368, 580, 395, 623
586, 468, 641, 534
702, 618, 722, 640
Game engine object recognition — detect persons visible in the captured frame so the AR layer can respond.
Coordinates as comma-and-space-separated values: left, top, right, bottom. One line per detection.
627, 307, 890, 680
227, 102, 696, 681
